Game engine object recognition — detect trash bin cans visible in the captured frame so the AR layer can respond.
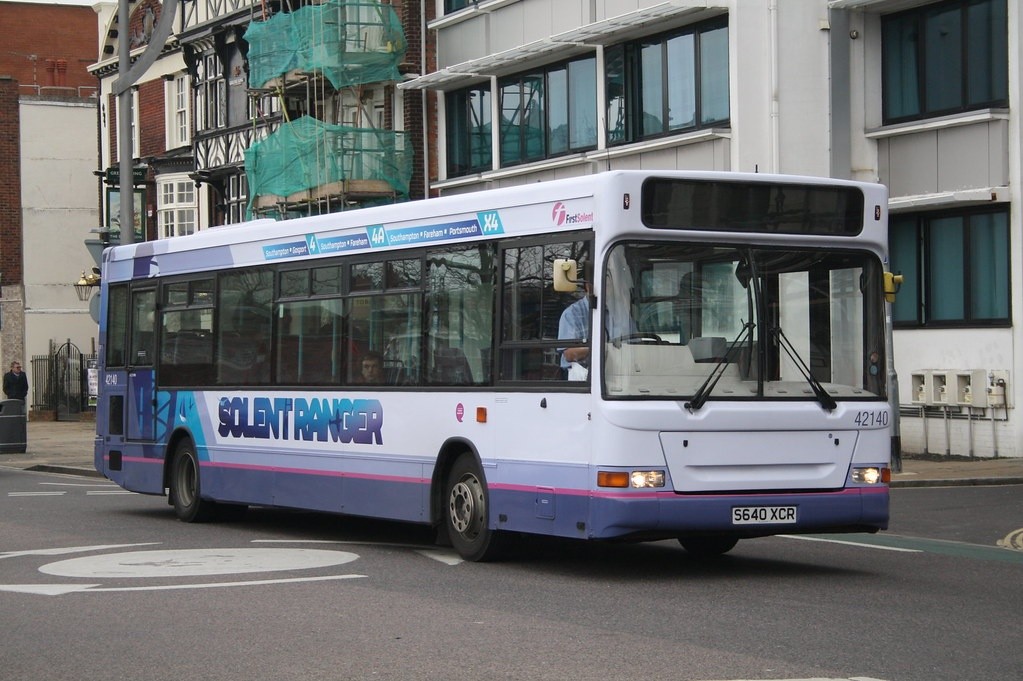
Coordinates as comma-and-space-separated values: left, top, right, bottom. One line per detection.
0, 399, 27, 454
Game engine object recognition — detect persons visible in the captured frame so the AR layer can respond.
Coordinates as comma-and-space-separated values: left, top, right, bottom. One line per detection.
354, 351, 390, 386
2, 361, 29, 400
557, 279, 641, 381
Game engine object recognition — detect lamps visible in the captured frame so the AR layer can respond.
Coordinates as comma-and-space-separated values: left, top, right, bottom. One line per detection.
74, 271, 98, 301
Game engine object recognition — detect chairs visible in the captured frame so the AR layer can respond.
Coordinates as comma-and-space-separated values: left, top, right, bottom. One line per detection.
134, 324, 354, 388
531, 278, 581, 383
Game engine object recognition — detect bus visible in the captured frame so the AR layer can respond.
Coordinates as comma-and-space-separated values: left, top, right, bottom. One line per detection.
93, 167, 906, 566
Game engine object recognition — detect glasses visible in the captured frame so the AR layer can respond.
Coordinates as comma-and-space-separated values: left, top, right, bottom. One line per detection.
11, 366, 21, 369
